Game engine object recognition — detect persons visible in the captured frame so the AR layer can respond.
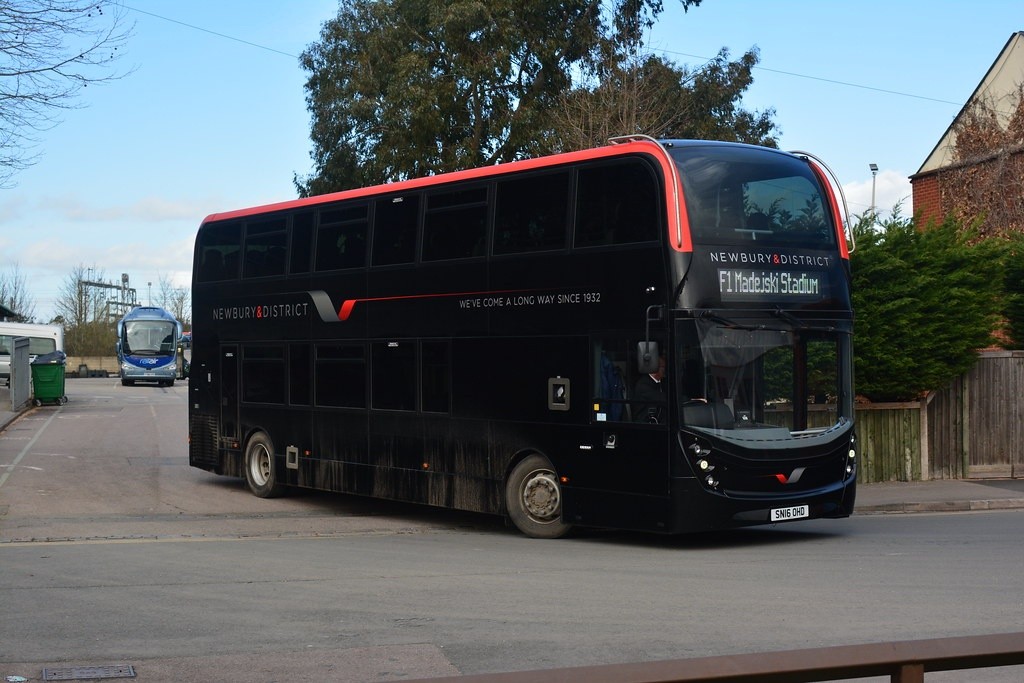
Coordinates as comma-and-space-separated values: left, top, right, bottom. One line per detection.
630, 356, 706, 424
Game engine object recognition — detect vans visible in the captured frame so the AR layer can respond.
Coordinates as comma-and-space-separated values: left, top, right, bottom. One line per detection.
0, 322, 64, 388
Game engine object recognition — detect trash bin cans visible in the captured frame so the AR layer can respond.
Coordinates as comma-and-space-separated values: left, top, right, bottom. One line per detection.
30, 361, 68, 407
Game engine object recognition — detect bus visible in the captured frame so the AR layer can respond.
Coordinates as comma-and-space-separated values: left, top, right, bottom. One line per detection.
176, 333, 192, 380
116, 306, 182, 387
189, 134, 856, 539
